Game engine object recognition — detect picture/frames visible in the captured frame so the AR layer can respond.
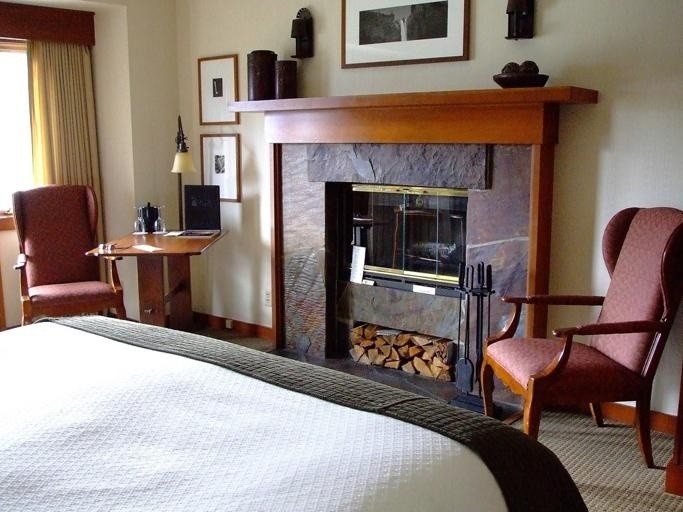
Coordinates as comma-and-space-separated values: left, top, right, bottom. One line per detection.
196, 53, 239, 126
339, 0, 471, 69
198, 132, 243, 205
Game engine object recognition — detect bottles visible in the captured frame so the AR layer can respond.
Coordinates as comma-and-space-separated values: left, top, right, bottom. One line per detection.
246, 49, 278, 101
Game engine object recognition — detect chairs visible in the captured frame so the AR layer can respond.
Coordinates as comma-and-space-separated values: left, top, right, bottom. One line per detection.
476, 205, 683, 469
10, 185, 127, 318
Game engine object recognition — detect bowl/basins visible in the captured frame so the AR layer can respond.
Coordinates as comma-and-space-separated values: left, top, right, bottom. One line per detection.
492, 74, 549, 89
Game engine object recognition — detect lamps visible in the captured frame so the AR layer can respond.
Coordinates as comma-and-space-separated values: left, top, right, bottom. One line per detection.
285, 7, 316, 58
502, 0, 535, 41
168, 113, 193, 229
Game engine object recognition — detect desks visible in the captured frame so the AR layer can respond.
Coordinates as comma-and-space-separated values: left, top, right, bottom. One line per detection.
79, 229, 231, 331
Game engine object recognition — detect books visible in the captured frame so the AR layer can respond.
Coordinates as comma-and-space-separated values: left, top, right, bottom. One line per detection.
177, 229, 220, 240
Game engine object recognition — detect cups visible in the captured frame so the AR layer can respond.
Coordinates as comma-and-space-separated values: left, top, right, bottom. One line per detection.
276, 59, 297, 98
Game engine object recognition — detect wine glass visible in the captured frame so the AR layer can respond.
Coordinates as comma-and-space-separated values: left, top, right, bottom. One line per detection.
132, 206, 146, 233
153, 205, 165, 233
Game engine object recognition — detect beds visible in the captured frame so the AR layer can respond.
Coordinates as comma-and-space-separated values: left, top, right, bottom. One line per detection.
0, 312, 586, 512
